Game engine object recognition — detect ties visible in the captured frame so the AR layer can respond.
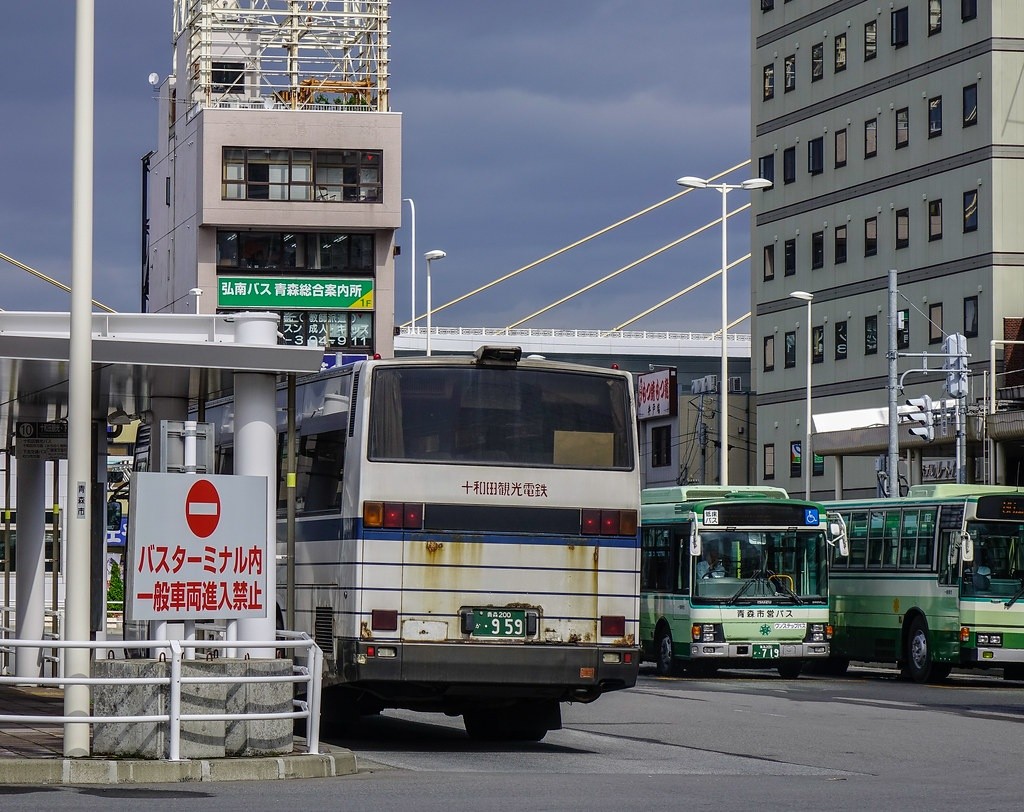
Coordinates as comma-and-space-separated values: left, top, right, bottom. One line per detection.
709, 566, 713, 578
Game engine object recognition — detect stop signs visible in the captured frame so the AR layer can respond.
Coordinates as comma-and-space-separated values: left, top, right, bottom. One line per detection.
186, 480, 221, 539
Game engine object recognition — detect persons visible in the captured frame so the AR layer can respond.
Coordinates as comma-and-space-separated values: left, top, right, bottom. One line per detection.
965, 558, 991, 581
698, 549, 725, 579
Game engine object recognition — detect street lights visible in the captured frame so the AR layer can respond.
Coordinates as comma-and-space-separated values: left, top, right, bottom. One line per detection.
788, 290, 814, 503
424, 249, 447, 357
676, 176, 772, 486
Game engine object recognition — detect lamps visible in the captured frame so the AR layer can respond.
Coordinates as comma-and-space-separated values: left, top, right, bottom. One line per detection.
648, 364, 677, 371
189, 288, 203, 315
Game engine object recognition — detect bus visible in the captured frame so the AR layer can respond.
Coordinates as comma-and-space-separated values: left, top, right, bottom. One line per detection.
639, 484, 849, 679
107, 347, 643, 745
775, 484, 1024, 684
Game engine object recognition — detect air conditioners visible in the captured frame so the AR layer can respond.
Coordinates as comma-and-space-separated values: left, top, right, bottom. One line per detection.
690, 375, 742, 395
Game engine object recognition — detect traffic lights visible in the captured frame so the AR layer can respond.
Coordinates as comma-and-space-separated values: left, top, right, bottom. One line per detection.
906, 395, 935, 443
